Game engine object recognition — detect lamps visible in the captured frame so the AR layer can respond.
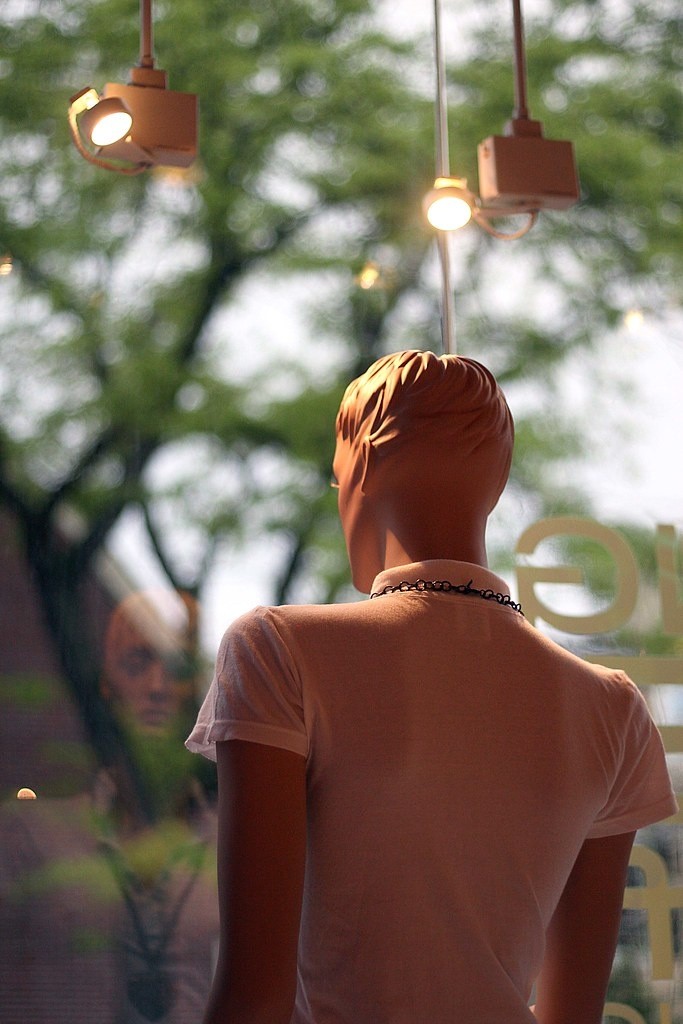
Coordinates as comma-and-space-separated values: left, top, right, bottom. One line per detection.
420, 0, 584, 242
65, 0, 203, 175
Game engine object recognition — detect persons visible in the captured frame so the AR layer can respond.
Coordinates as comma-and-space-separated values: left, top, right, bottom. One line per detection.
0, 587, 221, 1024
183, 347, 678, 1024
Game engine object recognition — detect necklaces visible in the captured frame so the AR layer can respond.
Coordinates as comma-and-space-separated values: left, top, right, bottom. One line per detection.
370, 577, 523, 615
101, 839, 207, 1018
